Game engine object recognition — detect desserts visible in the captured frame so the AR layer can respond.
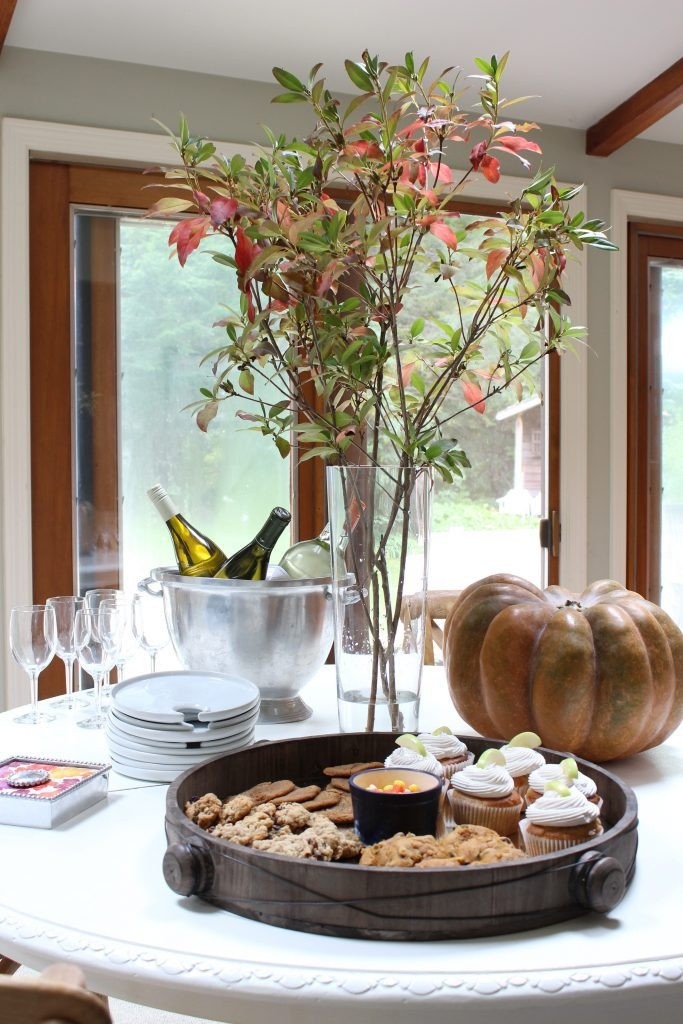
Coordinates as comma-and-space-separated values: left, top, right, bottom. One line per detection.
384, 726, 604, 856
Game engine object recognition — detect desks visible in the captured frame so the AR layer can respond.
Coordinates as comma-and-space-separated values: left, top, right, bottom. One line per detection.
0, 664, 681, 1024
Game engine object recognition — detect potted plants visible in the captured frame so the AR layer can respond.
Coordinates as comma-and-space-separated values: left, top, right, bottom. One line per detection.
153, 45, 640, 943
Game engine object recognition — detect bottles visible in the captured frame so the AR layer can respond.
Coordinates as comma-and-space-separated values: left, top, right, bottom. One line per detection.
273, 498, 366, 579
211, 506, 292, 579
145, 485, 229, 578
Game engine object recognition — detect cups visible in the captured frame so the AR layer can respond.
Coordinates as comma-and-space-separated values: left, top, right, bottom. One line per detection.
349, 769, 444, 843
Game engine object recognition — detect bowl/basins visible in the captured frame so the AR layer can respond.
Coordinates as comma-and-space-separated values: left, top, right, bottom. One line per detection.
140, 566, 368, 726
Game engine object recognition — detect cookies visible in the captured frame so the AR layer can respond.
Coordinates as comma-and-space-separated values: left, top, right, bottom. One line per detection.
185, 761, 527, 867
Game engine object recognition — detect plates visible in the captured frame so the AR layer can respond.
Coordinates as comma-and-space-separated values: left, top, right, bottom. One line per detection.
104, 671, 265, 786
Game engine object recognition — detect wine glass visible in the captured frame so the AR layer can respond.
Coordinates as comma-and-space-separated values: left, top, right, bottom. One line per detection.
10, 587, 172, 731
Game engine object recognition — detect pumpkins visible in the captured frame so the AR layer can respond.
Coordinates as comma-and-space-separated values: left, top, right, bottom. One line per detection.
443, 573, 682, 764
